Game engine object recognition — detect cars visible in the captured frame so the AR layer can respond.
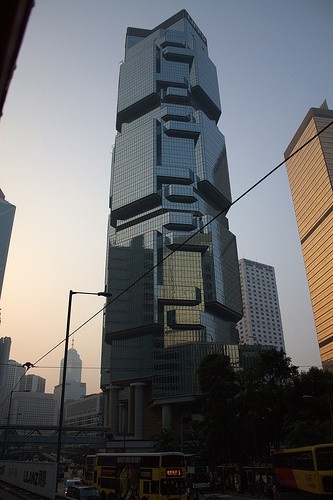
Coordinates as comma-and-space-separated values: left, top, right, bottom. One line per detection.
59, 473, 105, 500
216, 465, 277, 490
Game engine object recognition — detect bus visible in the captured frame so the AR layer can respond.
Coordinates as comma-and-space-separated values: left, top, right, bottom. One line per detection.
269, 442, 332, 497
185, 452, 208, 492
92, 450, 190, 500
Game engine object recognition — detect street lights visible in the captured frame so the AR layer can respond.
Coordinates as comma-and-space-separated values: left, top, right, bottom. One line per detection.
303, 394, 333, 420
53, 289, 114, 495
